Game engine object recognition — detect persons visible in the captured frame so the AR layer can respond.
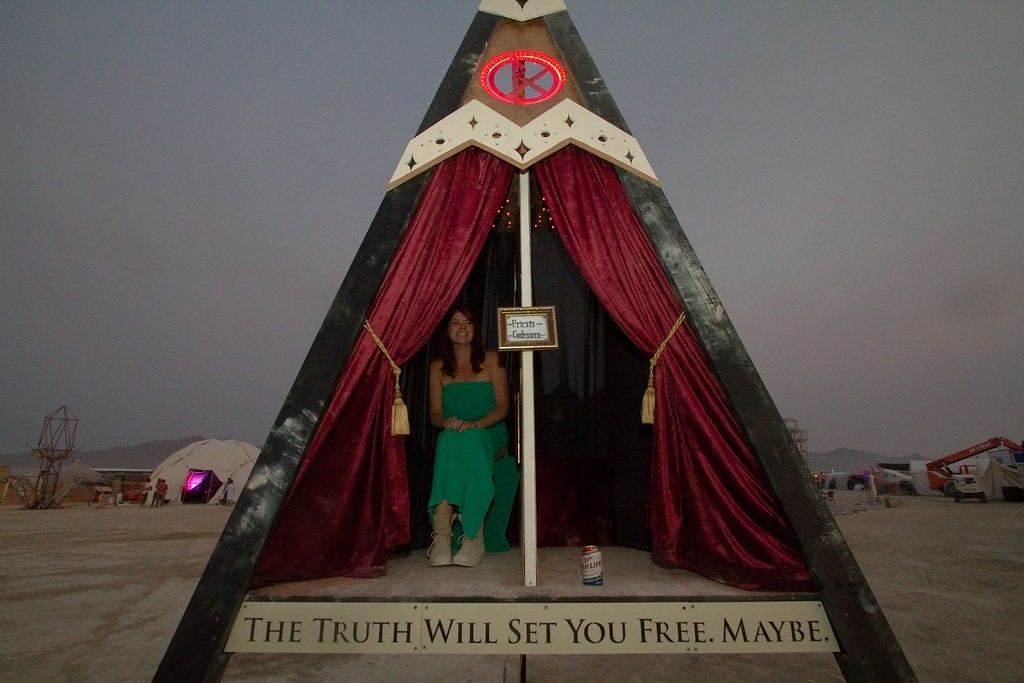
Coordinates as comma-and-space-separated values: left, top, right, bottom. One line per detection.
156, 479, 169, 507
140, 478, 152, 507
225, 479, 234, 505
222, 478, 231, 505
151, 478, 161, 507
426, 306, 509, 567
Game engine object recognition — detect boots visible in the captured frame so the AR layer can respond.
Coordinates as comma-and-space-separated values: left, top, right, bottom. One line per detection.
453, 515, 484, 566
428, 512, 457, 565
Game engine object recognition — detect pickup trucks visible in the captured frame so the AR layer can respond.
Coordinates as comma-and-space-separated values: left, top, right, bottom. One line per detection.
954, 473, 987, 504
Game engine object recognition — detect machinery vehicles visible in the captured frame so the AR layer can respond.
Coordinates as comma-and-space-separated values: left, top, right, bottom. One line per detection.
925, 435, 1024, 498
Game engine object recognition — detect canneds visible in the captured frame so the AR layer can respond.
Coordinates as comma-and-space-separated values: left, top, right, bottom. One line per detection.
581, 545, 604, 586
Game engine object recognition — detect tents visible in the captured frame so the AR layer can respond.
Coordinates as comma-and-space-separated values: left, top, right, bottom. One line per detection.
141, 439, 264, 505
